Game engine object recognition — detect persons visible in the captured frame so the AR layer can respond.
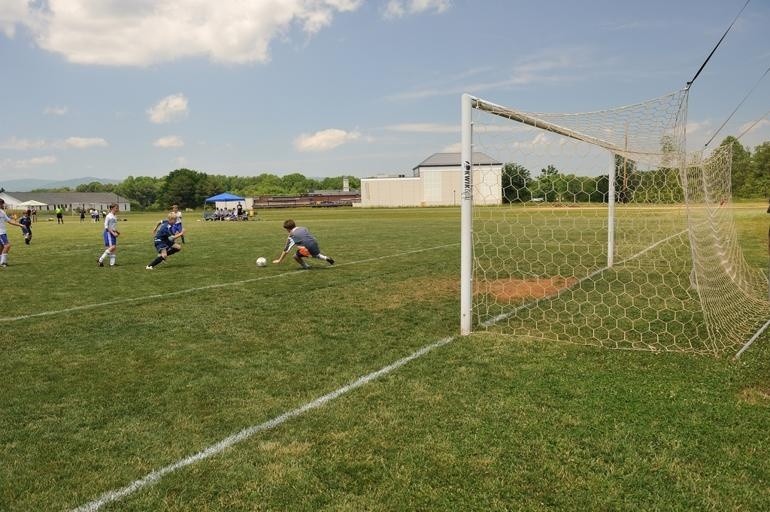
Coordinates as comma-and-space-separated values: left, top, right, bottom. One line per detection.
79, 207, 111, 224
0, 198, 27, 268
170, 204, 185, 244
55, 205, 65, 224
272, 218, 336, 270
31, 208, 37, 223
204, 201, 249, 222
96, 202, 122, 267
19, 209, 33, 245
145, 212, 184, 271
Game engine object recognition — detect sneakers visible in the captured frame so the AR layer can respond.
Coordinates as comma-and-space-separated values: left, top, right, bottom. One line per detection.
326, 257, 335, 265
0, 263, 8, 268
146, 265, 153, 270
97, 260, 118, 267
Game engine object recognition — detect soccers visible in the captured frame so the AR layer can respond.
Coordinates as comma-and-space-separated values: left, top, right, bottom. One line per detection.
256, 257, 267, 267
